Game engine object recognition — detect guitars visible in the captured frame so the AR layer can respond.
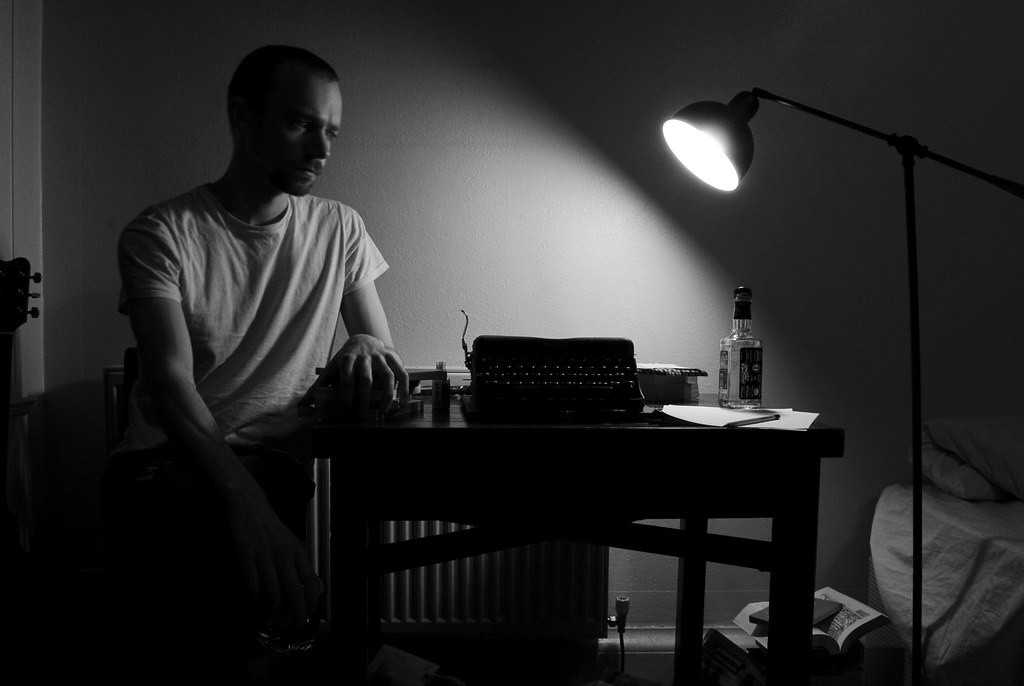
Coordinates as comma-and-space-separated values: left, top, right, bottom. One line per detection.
0, 255, 51, 686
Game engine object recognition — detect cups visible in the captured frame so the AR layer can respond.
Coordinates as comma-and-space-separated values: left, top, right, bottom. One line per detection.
255, 575, 328, 656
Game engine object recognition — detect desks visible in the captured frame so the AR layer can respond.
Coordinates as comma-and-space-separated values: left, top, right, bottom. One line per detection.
314, 390, 847, 686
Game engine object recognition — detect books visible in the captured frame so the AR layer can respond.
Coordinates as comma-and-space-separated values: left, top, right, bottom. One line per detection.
730, 584, 891, 655
700, 627, 768, 686
807, 667, 864, 686
746, 637, 866, 676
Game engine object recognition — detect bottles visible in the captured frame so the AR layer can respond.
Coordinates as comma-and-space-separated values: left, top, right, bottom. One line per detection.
719, 287, 764, 409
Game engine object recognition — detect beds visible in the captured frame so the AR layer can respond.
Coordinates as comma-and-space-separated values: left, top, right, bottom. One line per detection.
864, 481, 1024, 686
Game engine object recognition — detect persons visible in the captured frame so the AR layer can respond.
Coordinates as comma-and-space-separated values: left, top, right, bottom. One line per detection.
90, 43, 410, 686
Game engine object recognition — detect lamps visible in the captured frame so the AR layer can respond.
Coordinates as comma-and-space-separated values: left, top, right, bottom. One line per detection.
663, 87, 1024, 686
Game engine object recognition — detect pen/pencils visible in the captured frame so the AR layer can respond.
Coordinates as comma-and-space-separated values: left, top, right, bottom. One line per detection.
723, 414, 780, 428
